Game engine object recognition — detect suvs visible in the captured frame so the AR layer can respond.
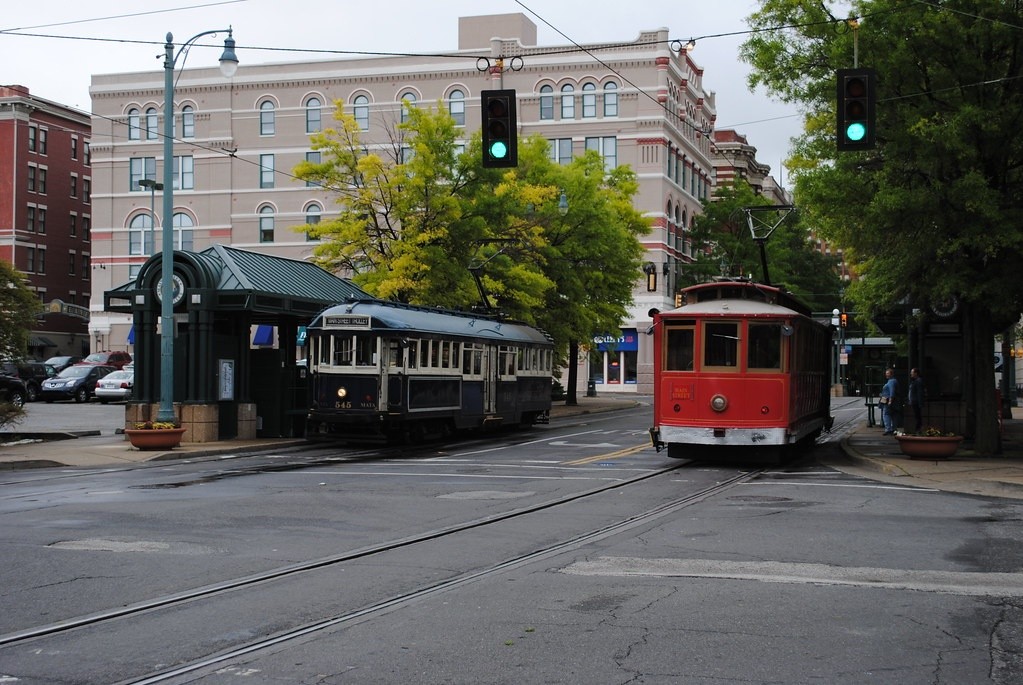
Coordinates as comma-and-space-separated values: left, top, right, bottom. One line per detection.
73, 350, 132, 370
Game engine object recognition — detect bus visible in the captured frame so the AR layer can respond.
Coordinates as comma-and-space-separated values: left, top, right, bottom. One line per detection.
305, 297, 556, 443
652, 280, 835, 464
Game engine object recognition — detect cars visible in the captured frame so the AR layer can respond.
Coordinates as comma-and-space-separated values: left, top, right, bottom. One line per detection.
0, 374, 28, 411
95, 371, 134, 405
39, 365, 117, 404
44, 356, 85, 374
0, 364, 59, 402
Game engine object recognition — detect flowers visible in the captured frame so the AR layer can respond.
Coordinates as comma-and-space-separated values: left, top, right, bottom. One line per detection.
131, 419, 182, 430
906, 424, 955, 438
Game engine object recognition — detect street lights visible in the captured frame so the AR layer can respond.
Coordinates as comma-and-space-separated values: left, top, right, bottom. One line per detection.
139, 179, 163, 258
94, 331, 100, 353
156, 24, 239, 423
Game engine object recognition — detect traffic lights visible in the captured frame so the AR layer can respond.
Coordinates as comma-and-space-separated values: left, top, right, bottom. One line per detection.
837, 67, 877, 151
481, 89, 518, 169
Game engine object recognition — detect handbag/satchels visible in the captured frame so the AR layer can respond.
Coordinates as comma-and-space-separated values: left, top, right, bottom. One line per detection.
880, 396, 888, 403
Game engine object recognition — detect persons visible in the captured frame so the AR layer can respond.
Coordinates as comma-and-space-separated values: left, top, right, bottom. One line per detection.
908, 368, 922, 432
880, 369, 897, 435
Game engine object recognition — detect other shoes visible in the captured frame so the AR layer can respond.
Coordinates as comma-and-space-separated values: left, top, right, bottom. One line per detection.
891, 431, 893, 434
883, 430, 891, 436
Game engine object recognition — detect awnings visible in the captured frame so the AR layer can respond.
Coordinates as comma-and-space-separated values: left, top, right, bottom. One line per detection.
297, 327, 307, 345
253, 325, 273, 345
127, 326, 133, 345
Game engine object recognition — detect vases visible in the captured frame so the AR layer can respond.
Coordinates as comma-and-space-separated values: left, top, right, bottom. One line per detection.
895, 435, 966, 461
125, 429, 185, 451
